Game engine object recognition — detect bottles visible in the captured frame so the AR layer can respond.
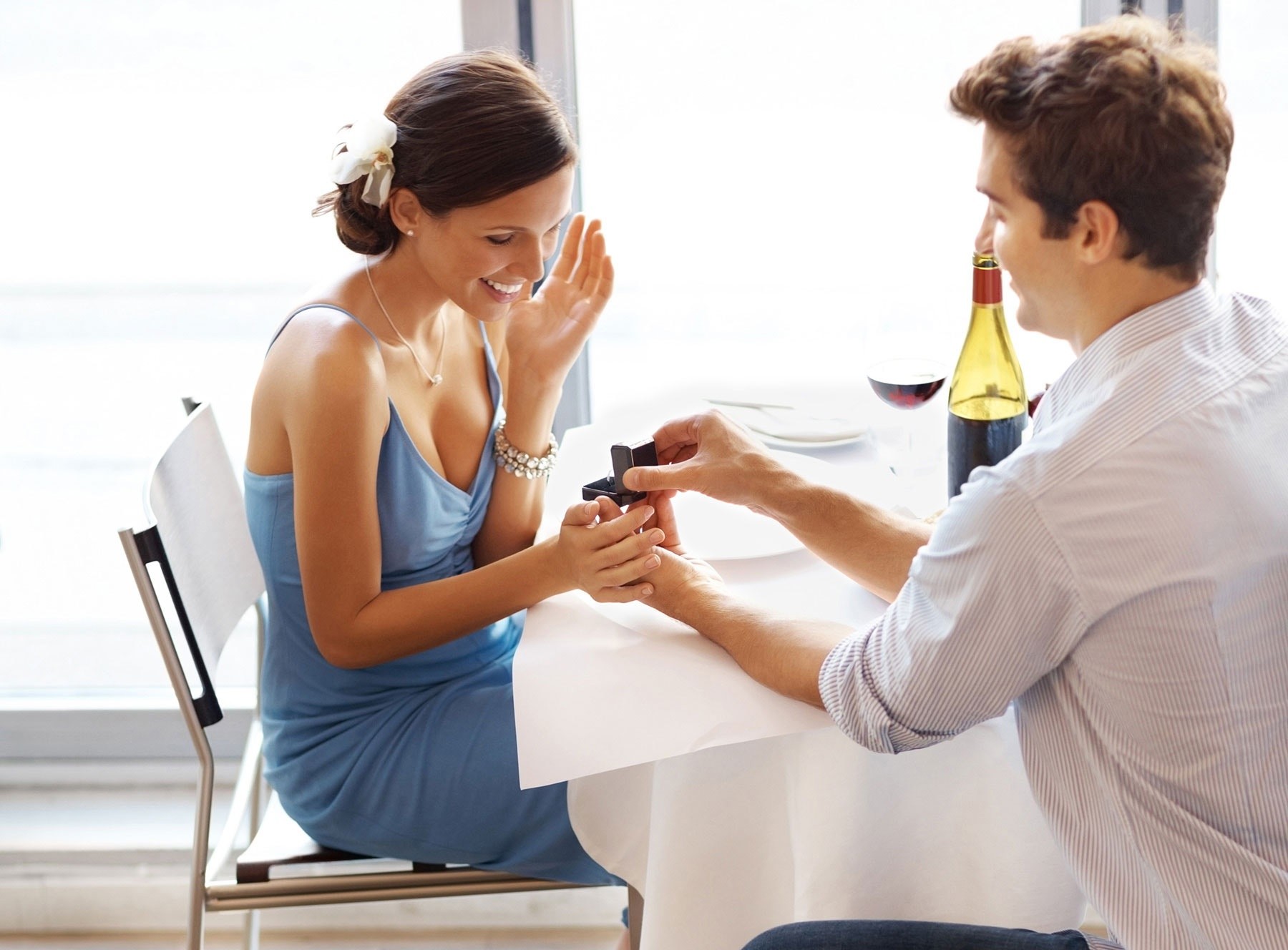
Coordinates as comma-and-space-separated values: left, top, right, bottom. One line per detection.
947, 250, 1030, 506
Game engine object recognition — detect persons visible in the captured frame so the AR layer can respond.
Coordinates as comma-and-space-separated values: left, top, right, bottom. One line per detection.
599, 22, 1288, 950
242, 49, 667, 948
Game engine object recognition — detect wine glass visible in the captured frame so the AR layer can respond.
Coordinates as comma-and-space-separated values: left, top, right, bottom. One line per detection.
859, 352, 952, 451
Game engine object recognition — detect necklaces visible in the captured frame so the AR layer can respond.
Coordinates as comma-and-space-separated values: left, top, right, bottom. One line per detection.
363, 255, 446, 386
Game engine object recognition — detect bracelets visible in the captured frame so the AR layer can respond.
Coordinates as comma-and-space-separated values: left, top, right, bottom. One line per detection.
492, 421, 559, 481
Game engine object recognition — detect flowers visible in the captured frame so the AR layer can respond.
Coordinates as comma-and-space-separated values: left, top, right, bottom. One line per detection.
331, 109, 398, 210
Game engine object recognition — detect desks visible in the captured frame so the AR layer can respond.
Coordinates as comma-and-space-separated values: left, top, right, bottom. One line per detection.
510, 415, 1094, 950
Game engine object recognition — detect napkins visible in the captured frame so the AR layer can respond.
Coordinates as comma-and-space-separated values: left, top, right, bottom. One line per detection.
704, 396, 862, 440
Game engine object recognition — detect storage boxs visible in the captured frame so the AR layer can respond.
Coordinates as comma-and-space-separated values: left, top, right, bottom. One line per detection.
582, 433, 660, 507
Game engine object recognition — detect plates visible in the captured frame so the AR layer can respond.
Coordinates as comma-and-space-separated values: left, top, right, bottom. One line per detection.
741, 418, 871, 449
669, 447, 855, 563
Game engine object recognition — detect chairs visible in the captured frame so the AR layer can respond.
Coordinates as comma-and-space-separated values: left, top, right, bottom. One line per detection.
116, 396, 644, 950
